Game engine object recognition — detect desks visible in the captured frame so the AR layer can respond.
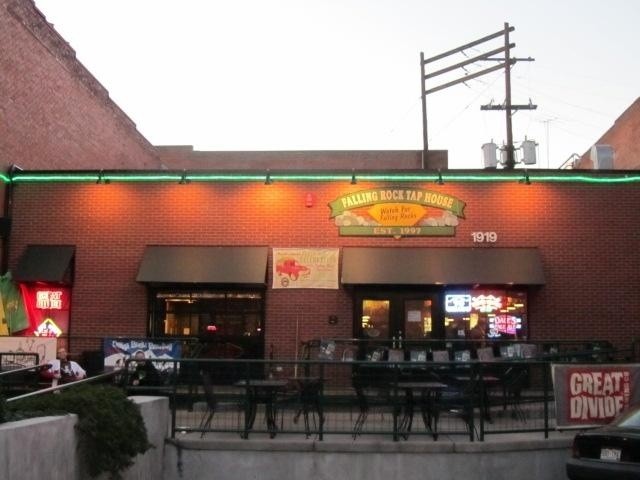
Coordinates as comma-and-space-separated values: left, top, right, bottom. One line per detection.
230, 376, 292, 439
386, 378, 446, 441
448, 368, 509, 422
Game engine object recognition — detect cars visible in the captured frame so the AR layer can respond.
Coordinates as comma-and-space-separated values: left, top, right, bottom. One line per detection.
565, 404, 640, 480
0, 359, 57, 398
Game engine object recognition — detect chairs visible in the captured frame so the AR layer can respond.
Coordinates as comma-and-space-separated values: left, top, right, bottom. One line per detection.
437, 361, 487, 442
197, 369, 255, 440
350, 371, 410, 444
276, 376, 325, 441
495, 356, 529, 423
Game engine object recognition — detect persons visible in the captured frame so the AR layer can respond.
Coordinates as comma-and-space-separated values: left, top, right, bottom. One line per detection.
443, 316, 489, 347
47, 347, 86, 391
129, 350, 160, 388
